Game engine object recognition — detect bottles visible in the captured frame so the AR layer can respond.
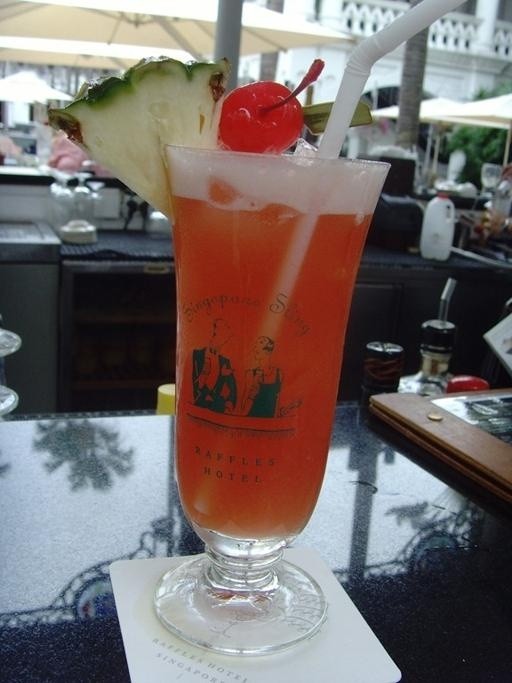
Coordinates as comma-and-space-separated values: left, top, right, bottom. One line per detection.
352, 317, 492, 405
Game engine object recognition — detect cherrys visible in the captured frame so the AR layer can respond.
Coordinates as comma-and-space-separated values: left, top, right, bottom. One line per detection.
219, 59, 325, 154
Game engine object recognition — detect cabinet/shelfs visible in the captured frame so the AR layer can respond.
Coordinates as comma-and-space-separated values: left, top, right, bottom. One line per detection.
337, 272, 512, 401
57, 260, 176, 412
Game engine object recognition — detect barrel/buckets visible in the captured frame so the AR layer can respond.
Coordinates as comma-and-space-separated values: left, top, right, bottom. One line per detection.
420, 191, 456, 258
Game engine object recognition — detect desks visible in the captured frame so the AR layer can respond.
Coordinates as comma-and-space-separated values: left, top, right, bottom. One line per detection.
0, 403, 512, 683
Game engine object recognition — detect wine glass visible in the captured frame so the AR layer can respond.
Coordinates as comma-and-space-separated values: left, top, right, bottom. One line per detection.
148, 135, 392, 660
47, 169, 106, 232
